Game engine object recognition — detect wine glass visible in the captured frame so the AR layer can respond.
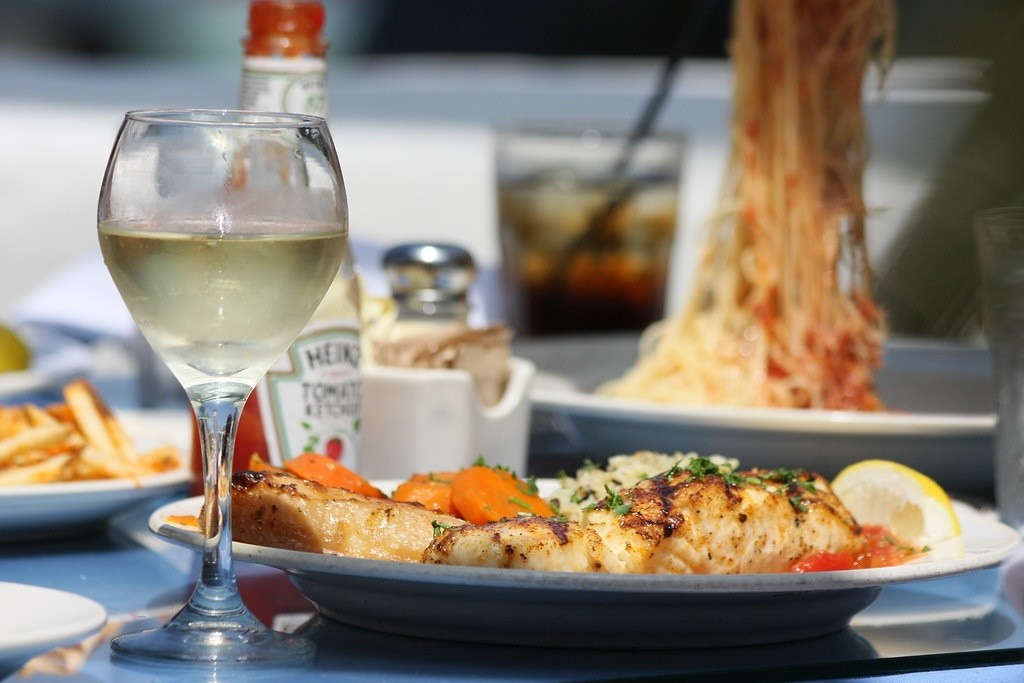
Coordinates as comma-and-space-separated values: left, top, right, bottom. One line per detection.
97, 106, 348, 667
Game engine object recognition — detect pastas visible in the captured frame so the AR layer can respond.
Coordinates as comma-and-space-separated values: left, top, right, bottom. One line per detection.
594, 0, 898, 408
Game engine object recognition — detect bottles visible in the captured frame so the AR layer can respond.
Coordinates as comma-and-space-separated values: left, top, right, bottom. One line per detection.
188, 1, 351, 491
362, 241, 515, 404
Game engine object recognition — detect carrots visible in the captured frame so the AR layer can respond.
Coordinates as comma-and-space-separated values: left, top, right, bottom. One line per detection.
250, 451, 558, 526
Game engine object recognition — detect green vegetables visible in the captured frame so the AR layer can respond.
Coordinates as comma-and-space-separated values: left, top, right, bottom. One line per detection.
466, 454, 816, 514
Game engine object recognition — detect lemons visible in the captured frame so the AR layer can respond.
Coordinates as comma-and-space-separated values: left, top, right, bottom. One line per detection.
832, 458, 961, 545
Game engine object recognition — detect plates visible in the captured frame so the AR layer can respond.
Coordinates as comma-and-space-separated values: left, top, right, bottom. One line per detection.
510, 330, 1004, 491
0, 581, 109, 670
0, 403, 199, 535
146, 472, 1019, 648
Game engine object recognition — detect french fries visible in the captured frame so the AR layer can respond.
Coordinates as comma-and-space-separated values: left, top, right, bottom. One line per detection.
0, 377, 181, 485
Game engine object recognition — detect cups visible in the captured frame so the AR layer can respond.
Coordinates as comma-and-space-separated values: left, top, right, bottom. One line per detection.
357, 332, 537, 483
495, 127, 686, 339
